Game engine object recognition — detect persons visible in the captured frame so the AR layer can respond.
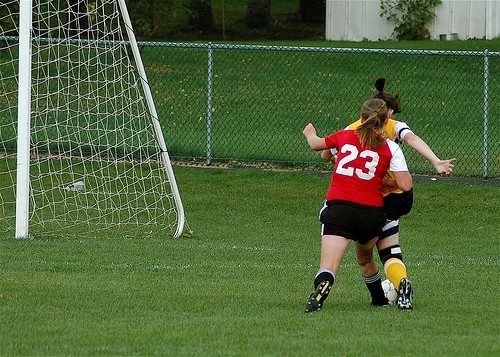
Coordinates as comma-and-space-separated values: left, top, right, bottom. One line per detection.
321, 77, 456, 312
301, 98, 415, 315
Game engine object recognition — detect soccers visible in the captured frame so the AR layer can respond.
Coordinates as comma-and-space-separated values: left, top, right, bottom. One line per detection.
380, 278, 399, 306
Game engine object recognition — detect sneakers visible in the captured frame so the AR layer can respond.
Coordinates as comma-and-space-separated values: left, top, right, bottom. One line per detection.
305, 280, 333, 312
397, 279, 413, 311
